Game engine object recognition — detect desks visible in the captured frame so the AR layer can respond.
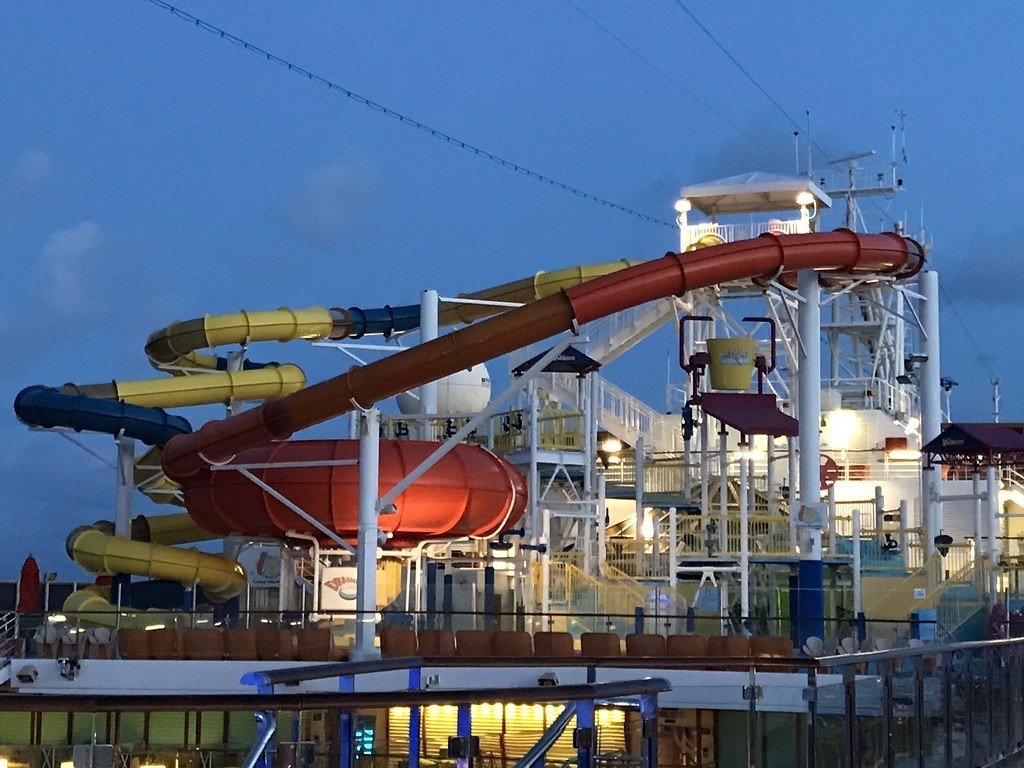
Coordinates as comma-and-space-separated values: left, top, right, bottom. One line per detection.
118, 750, 155, 768
38, 745, 73, 768
188, 747, 243, 768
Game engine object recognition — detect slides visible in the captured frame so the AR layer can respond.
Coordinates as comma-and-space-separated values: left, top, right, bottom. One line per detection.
11, 229, 927, 632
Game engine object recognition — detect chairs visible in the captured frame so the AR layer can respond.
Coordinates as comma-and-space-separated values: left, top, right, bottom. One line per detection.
117, 624, 337, 661
381, 628, 798, 674
